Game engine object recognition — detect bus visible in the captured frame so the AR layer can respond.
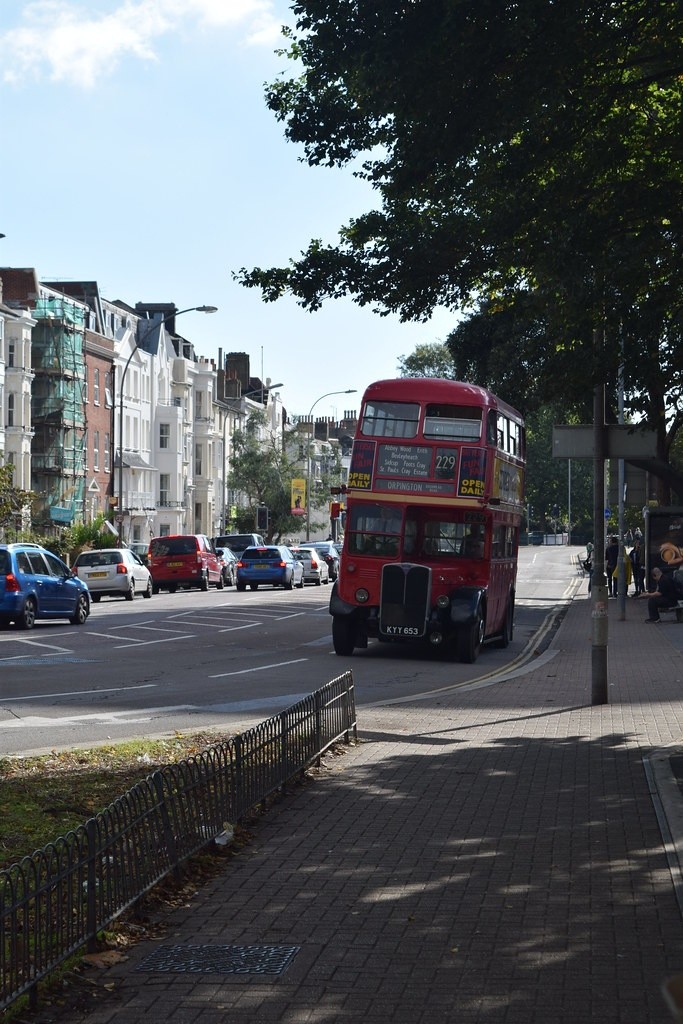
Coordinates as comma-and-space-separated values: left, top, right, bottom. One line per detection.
328, 380, 529, 663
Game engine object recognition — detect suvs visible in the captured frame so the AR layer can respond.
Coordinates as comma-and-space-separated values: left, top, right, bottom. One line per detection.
208, 534, 268, 562
146, 534, 228, 594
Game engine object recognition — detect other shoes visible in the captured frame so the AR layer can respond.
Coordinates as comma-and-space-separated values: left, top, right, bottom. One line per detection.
632, 592, 641, 597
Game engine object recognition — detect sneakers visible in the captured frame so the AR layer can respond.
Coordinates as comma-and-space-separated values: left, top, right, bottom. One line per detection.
613, 595, 618, 599
645, 619, 661, 624
608, 595, 613, 599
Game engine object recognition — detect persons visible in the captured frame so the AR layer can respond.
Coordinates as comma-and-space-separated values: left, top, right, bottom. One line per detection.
638, 568, 678, 624
460, 523, 484, 559
364, 507, 402, 551
325, 532, 344, 545
285, 539, 293, 547
588, 528, 645, 599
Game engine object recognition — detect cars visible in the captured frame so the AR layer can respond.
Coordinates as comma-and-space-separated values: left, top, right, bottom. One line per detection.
123, 544, 151, 569
71, 548, 154, 603
0, 542, 93, 631
236, 543, 306, 589
301, 541, 345, 582
213, 546, 240, 586
285, 546, 330, 586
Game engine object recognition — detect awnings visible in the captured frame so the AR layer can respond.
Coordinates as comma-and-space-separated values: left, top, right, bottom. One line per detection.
114, 450, 159, 471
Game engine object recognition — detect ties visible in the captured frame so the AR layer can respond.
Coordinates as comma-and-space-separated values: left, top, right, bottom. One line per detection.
383, 520, 387, 542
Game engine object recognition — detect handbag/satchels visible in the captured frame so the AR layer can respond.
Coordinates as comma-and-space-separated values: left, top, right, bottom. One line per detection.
612, 550, 631, 585
583, 559, 592, 572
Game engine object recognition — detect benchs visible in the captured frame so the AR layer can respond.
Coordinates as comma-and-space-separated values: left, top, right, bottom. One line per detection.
659, 600, 683, 622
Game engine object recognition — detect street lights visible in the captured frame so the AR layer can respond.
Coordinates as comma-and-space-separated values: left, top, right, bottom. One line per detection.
117, 303, 220, 544
220, 383, 285, 534
305, 389, 359, 538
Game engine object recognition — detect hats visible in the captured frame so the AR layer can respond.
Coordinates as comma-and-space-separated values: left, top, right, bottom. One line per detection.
588, 542, 593, 550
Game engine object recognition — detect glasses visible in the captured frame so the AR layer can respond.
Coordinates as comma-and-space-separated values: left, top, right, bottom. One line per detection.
652, 573, 657, 578
